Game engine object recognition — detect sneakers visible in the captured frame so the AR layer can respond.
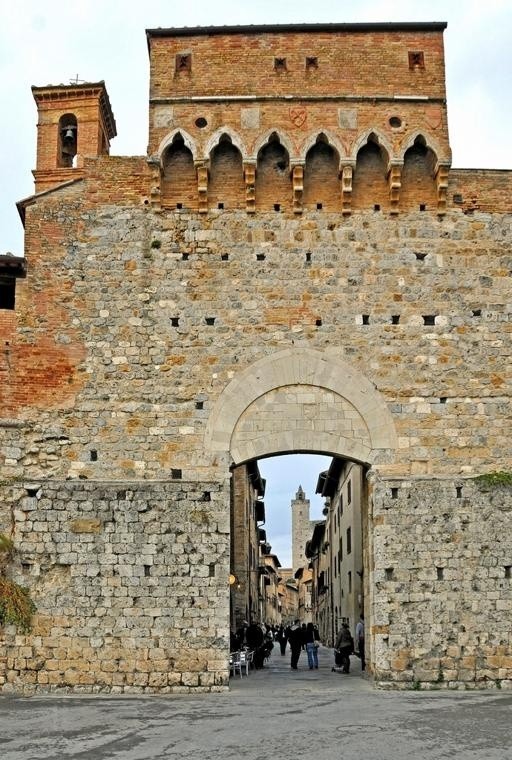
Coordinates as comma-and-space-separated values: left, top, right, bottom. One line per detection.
291, 665, 318, 669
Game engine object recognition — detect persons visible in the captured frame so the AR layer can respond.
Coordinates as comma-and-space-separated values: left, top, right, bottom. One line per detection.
231, 619, 321, 669
356, 613, 364, 670
335, 623, 353, 673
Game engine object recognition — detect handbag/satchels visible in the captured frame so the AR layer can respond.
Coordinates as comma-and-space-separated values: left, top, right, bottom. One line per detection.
314, 640, 319, 649
